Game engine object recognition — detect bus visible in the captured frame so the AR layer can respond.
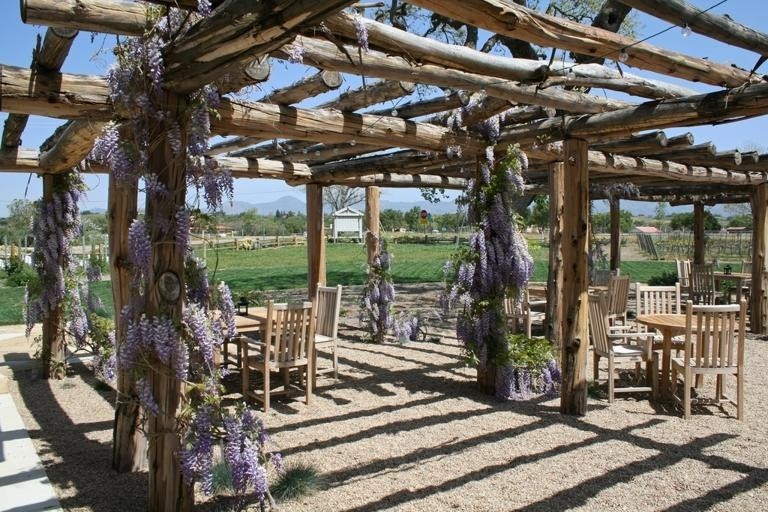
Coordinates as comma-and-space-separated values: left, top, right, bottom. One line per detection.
202, 229, 227, 238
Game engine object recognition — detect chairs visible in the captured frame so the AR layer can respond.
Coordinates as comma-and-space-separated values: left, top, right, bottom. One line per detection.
585, 259, 753, 421
208, 284, 343, 413
504, 291, 548, 340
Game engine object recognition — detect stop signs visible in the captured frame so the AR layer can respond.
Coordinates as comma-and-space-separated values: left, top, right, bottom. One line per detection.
420, 210, 426, 218
